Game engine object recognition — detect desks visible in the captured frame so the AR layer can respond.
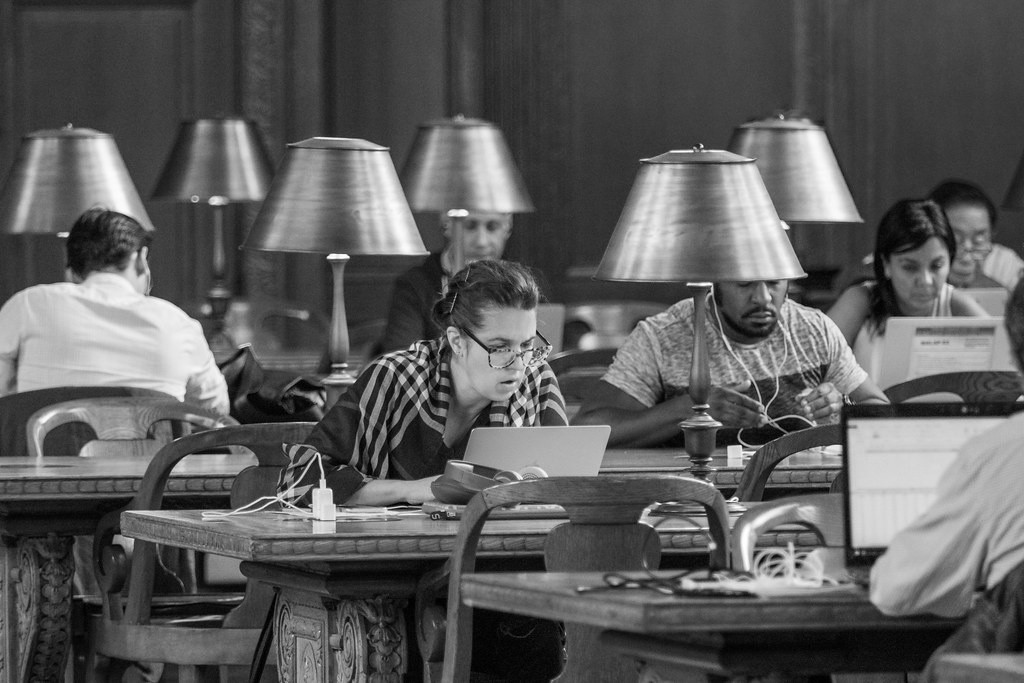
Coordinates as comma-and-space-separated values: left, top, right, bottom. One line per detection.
0, 450, 847, 683
461, 573, 961, 683
120, 502, 846, 683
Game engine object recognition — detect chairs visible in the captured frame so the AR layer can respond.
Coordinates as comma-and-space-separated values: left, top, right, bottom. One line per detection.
0, 302, 1024, 683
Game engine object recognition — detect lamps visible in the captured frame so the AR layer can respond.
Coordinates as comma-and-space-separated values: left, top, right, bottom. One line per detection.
591, 145, 809, 484
403, 116, 537, 278
725, 115, 865, 302
242, 137, 431, 413
0, 124, 154, 283
151, 117, 273, 352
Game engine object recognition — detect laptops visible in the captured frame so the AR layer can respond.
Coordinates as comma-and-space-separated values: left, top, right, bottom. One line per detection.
464, 424, 612, 477
880, 313, 1012, 393
839, 400, 1024, 587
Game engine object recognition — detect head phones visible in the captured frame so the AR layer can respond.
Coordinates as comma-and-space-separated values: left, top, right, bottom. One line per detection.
444, 460, 549, 508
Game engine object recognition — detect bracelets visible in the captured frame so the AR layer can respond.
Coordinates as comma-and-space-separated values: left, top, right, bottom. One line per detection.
844, 394, 856, 406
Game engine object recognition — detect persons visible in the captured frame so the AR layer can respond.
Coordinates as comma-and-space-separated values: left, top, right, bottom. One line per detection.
826, 198, 992, 406
278, 259, 569, 683
867, 274, 1024, 620
0, 209, 232, 601
862, 179, 1024, 294
569, 276, 890, 446
382, 209, 512, 351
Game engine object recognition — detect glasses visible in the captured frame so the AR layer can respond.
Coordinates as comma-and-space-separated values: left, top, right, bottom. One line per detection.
953, 246, 992, 262
459, 326, 554, 370
